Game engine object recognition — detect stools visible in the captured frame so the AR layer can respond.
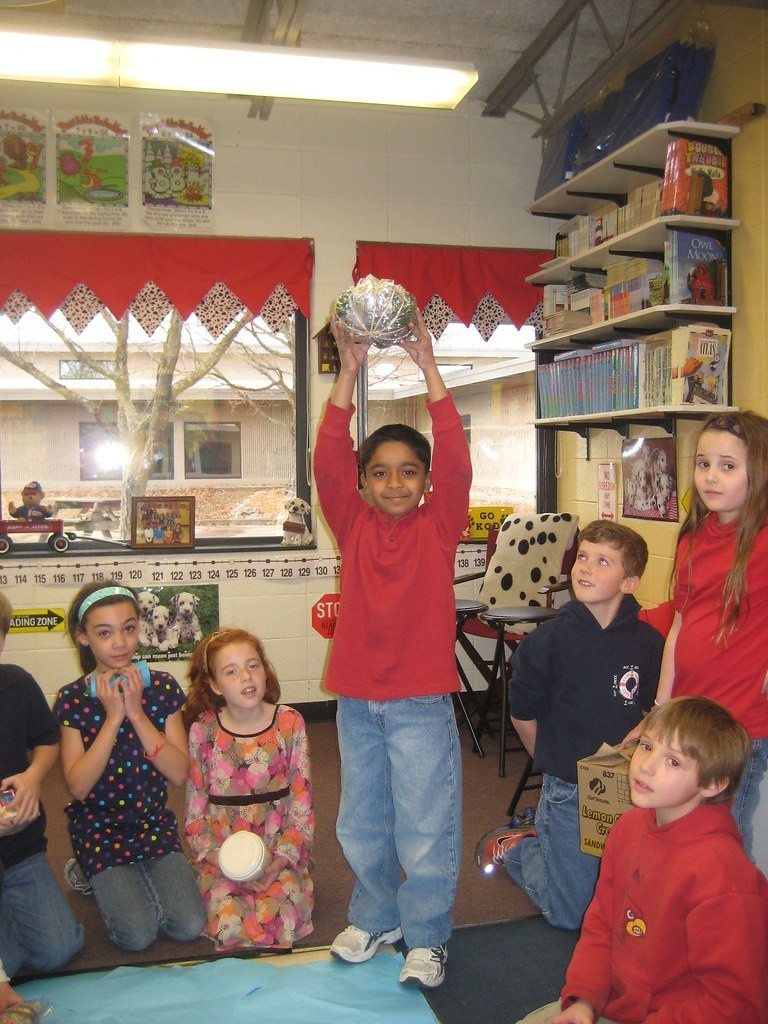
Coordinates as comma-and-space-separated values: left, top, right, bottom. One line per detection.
453, 598, 486, 758
475, 607, 559, 776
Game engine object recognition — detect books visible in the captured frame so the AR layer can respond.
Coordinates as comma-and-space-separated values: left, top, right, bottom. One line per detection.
538, 136, 731, 418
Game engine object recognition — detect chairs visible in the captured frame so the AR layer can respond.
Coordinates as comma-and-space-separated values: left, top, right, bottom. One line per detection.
453, 514, 582, 753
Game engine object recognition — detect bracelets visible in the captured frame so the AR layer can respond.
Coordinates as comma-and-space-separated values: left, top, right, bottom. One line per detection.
143, 732, 165, 761
654, 699, 661, 707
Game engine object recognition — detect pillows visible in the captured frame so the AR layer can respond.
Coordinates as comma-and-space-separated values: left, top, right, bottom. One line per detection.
478, 510, 579, 637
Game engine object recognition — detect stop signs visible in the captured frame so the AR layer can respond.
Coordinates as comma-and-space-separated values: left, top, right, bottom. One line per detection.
312, 594, 341, 639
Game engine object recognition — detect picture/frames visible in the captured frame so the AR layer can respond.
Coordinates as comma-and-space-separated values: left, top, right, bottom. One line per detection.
131, 496, 195, 548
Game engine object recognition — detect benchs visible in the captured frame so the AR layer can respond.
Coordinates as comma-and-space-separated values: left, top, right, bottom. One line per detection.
55, 518, 92, 525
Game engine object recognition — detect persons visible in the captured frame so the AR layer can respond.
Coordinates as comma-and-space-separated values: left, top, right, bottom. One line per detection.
313, 302, 473, 989
514, 695, 768, 1024
473, 518, 667, 928
54, 580, 206, 949
0, 960, 37, 1024
140, 501, 183, 544
1, 593, 86, 985
181, 627, 316, 951
652, 412, 768, 877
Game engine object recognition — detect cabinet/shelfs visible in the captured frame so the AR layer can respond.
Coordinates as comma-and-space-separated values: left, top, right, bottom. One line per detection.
524, 100, 761, 462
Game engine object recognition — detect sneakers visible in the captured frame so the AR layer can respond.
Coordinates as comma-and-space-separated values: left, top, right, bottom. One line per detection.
64, 858, 93, 895
398, 942, 448, 989
475, 807, 537, 876
330, 925, 402, 964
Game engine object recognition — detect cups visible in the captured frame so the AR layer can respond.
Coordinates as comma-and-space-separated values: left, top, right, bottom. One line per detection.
218, 831, 272, 881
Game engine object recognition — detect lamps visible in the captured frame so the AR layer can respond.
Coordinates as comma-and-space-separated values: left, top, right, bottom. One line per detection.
0, 21, 479, 110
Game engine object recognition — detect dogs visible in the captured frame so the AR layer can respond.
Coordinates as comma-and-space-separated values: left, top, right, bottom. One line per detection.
135, 591, 202, 652
281, 498, 314, 546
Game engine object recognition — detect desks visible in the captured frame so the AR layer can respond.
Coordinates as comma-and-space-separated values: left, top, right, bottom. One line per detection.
48, 497, 121, 539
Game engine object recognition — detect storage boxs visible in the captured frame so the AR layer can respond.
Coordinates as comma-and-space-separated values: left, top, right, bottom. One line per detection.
577, 740, 641, 858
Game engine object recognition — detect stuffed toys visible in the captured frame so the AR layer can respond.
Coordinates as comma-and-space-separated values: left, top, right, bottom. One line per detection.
8, 481, 53, 522
